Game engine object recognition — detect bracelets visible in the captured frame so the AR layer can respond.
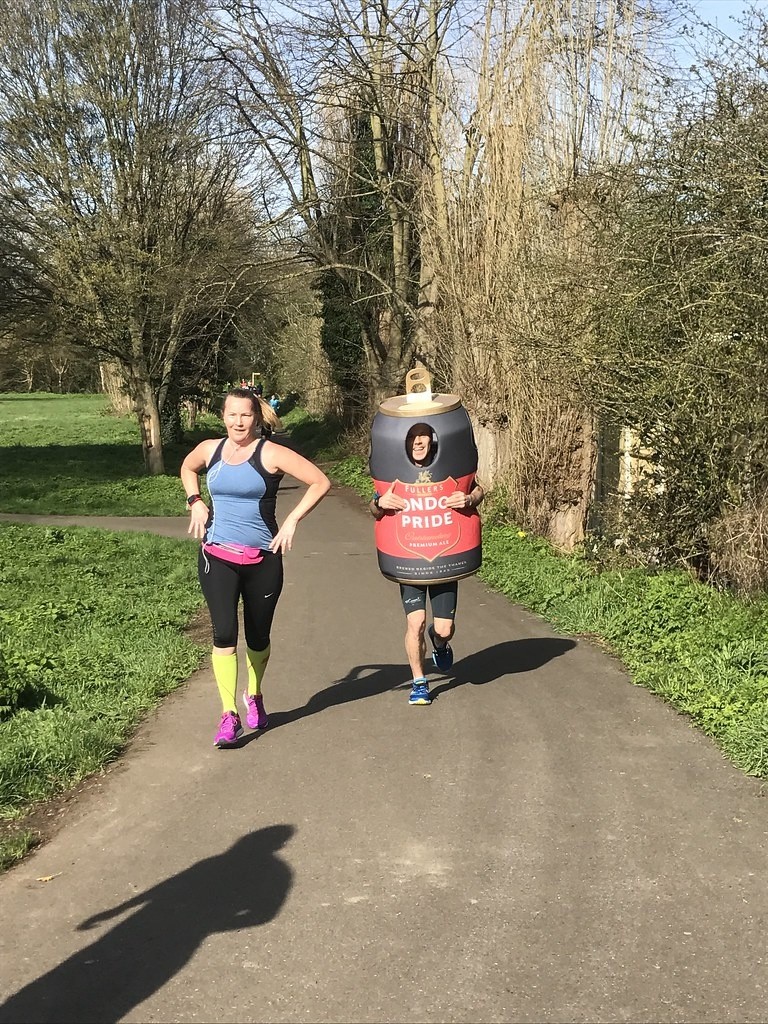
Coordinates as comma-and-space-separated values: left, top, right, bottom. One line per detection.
468, 495, 472, 508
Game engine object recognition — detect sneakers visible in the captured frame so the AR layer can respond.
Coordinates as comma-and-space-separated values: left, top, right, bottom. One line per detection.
407, 679, 433, 706
242, 689, 269, 729
426, 623, 454, 674
213, 709, 244, 746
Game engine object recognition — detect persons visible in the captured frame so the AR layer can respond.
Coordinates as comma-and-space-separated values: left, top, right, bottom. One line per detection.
180, 377, 333, 748
369, 424, 484, 705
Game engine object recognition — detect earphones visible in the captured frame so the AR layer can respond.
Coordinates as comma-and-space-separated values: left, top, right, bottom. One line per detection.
255, 420, 257, 426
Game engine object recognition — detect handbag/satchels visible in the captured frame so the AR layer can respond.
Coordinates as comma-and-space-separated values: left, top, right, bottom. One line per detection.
200, 538, 275, 565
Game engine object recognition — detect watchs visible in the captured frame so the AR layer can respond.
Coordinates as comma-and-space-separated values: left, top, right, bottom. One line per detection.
374, 495, 383, 510
186, 494, 203, 507
